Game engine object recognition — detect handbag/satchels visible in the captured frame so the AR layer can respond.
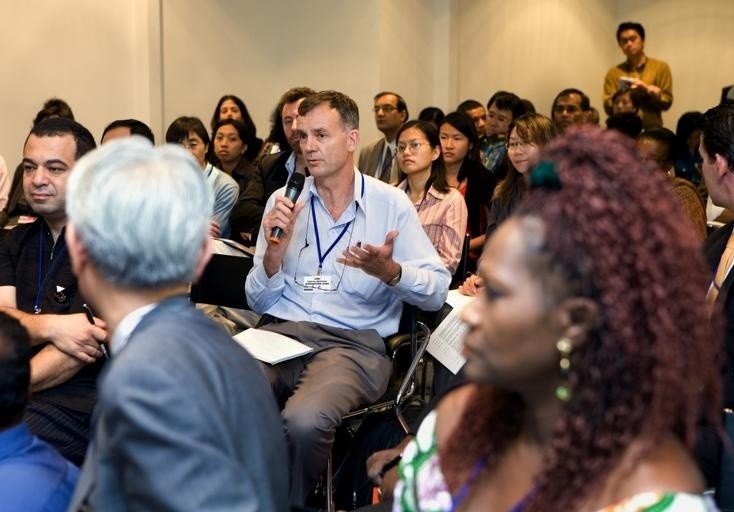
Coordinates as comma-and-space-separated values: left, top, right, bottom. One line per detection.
191, 252, 255, 312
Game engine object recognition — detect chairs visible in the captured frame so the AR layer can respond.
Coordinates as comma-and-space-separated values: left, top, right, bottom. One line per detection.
322, 231, 473, 512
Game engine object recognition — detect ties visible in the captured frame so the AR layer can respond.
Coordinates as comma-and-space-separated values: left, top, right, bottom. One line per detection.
704, 230, 734, 312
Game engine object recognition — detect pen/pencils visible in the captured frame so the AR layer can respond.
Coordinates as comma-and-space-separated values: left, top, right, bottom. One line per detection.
82, 303, 109, 360
353, 452, 403, 497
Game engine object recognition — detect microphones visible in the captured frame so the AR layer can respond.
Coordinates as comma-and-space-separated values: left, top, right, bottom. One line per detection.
268, 173, 305, 244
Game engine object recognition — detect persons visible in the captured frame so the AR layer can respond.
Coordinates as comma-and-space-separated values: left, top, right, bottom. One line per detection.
390, 124, 727, 511
0, 311, 83, 510
354, 367, 468, 511
64, 135, 291, 511
603, 22, 672, 134
1, 87, 734, 466
237, 91, 452, 511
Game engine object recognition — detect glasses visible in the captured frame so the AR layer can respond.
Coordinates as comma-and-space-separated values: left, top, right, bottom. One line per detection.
397, 141, 431, 152
295, 244, 349, 291
505, 140, 532, 151
372, 106, 399, 112
